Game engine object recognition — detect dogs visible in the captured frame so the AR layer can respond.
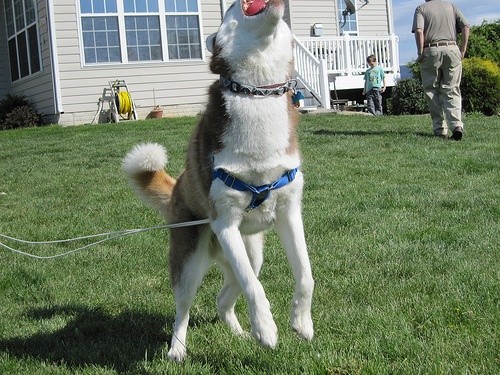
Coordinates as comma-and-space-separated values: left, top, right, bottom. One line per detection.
122, 0, 314, 362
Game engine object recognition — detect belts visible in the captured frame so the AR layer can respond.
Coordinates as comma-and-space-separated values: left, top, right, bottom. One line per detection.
423, 42, 459, 48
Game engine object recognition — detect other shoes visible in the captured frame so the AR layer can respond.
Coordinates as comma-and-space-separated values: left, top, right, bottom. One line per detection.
450, 126, 463, 141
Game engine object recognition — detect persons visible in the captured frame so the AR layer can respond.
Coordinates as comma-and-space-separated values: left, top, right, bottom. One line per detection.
412, 0, 471, 142
362, 55, 388, 116
